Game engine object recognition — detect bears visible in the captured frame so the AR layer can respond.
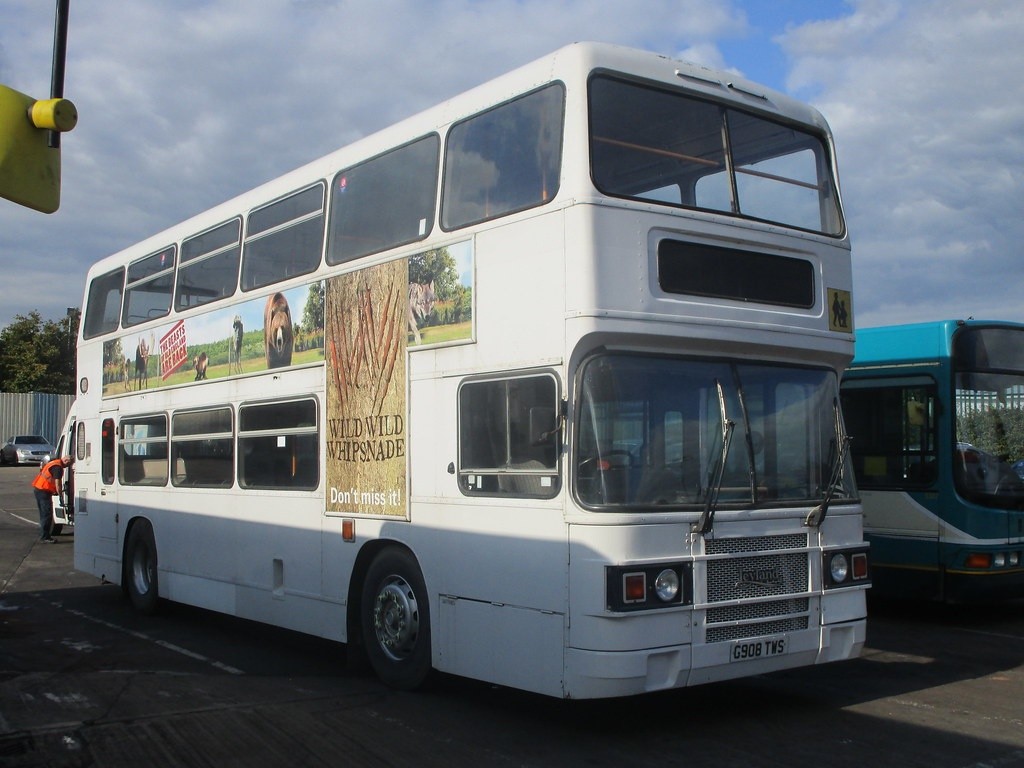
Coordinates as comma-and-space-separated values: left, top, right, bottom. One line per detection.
264, 293, 294, 369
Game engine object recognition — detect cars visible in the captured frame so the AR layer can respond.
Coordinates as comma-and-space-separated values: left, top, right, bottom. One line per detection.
0, 434, 56, 466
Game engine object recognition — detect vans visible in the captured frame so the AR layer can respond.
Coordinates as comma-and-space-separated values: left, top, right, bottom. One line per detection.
48, 398, 77, 536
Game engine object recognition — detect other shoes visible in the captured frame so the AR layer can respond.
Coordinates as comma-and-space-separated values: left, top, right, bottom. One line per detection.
40, 538, 57, 544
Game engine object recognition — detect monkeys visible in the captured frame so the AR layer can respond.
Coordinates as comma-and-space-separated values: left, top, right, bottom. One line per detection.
193, 352, 209, 381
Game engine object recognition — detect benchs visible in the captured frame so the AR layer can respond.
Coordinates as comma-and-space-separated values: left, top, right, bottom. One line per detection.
176, 457, 277, 486
222, 284, 235, 295
285, 264, 301, 277
107, 455, 167, 479
126, 314, 147, 324
254, 272, 274, 287
148, 308, 168, 318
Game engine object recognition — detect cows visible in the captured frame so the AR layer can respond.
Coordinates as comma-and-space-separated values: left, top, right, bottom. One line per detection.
134, 338, 149, 391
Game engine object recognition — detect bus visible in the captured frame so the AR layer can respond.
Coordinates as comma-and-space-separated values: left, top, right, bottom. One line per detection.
615, 315, 1023, 603
71, 38, 871, 701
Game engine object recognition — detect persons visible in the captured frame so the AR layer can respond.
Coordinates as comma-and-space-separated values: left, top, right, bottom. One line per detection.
32, 455, 75, 544
